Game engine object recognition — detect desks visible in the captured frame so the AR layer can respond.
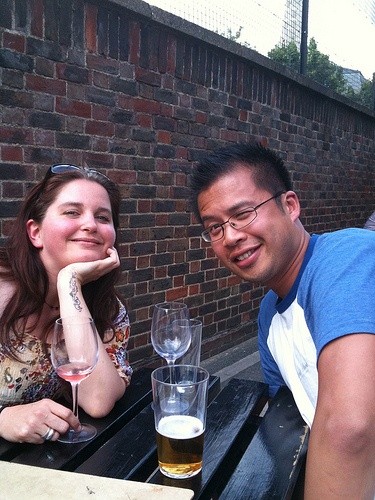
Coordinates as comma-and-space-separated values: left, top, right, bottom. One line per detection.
1, 368, 310, 500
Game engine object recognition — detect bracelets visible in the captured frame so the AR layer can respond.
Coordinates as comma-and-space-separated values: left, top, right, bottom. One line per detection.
0, 404, 7, 413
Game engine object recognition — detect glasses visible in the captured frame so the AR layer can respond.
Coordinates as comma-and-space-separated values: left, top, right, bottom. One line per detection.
38, 163, 115, 191
200, 192, 285, 243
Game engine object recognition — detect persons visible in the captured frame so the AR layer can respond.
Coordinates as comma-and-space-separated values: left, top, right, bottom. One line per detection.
363, 210, 375, 231
0, 164, 133, 444
187, 139, 375, 500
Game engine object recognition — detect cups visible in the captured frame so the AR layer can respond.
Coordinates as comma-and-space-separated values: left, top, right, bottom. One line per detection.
150, 364, 209, 480
172, 318, 202, 394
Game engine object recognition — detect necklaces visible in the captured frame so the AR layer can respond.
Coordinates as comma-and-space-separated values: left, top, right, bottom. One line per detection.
45, 301, 60, 311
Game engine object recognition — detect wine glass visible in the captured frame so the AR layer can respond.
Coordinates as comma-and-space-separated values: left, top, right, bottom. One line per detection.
50, 316, 98, 444
150, 302, 192, 413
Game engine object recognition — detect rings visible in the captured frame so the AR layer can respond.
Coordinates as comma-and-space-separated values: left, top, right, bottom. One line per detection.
41, 427, 55, 440
